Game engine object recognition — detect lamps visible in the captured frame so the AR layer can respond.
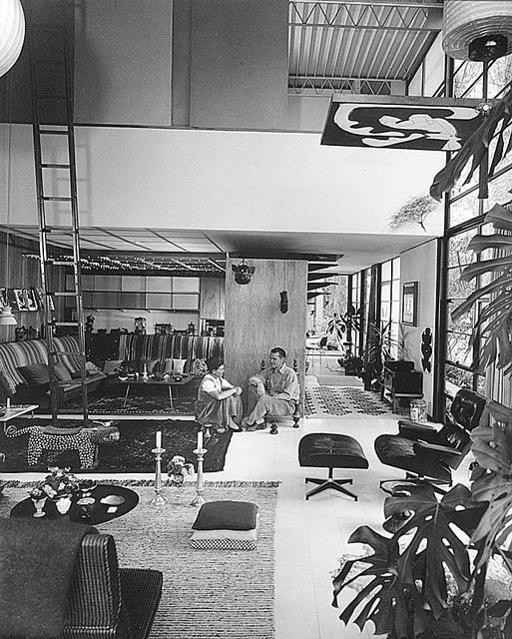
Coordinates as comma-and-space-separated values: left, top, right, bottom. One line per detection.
0, 52, 17, 325
441, 0, 512, 63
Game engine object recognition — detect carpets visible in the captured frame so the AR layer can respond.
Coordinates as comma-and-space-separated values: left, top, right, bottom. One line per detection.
316, 373, 364, 387
0, 478, 280, 638
0, 417, 232, 473
33, 383, 200, 417
304, 384, 410, 419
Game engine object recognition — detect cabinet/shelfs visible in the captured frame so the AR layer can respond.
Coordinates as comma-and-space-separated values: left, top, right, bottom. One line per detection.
64, 273, 201, 313
381, 366, 423, 413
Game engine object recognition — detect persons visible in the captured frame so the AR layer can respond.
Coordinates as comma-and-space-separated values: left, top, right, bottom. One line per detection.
196, 357, 244, 433
245, 346, 300, 430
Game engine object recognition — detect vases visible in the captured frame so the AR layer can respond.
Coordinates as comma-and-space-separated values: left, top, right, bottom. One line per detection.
54, 496, 71, 514
172, 474, 184, 487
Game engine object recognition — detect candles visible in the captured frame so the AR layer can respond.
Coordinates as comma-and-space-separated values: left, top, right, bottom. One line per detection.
155, 431, 161, 448
197, 431, 202, 452
144, 363, 146, 377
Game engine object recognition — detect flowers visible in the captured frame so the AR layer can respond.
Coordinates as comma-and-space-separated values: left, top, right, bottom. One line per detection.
40, 465, 78, 498
28, 487, 46, 501
167, 455, 195, 476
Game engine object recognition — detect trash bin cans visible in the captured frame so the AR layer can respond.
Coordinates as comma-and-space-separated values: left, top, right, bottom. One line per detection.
409, 400, 428, 424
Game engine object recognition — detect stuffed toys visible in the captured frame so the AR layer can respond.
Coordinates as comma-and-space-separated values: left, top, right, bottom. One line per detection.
165, 454, 194, 490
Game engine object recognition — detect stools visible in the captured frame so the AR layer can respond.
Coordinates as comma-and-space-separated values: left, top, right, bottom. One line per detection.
298, 433, 370, 502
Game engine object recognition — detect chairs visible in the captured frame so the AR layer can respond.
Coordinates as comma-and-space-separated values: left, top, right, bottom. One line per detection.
374, 387, 486, 501
258, 358, 300, 429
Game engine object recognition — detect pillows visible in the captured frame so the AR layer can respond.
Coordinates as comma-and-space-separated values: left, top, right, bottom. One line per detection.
23, 362, 58, 386
103, 359, 124, 375
165, 357, 187, 374
54, 362, 72, 382
137, 358, 160, 372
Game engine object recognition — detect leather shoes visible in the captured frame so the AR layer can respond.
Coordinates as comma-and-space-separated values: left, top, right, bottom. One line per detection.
227, 423, 242, 432
255, 422, 267, 429
241, 420, 257, 431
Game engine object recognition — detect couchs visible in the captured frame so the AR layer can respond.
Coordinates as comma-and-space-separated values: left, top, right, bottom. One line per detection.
108, 332, 224, 390
0, 518, 164, 639
0, 335, 107, 408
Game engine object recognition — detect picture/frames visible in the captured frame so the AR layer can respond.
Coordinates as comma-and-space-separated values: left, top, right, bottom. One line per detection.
402, 281, 418, 327
